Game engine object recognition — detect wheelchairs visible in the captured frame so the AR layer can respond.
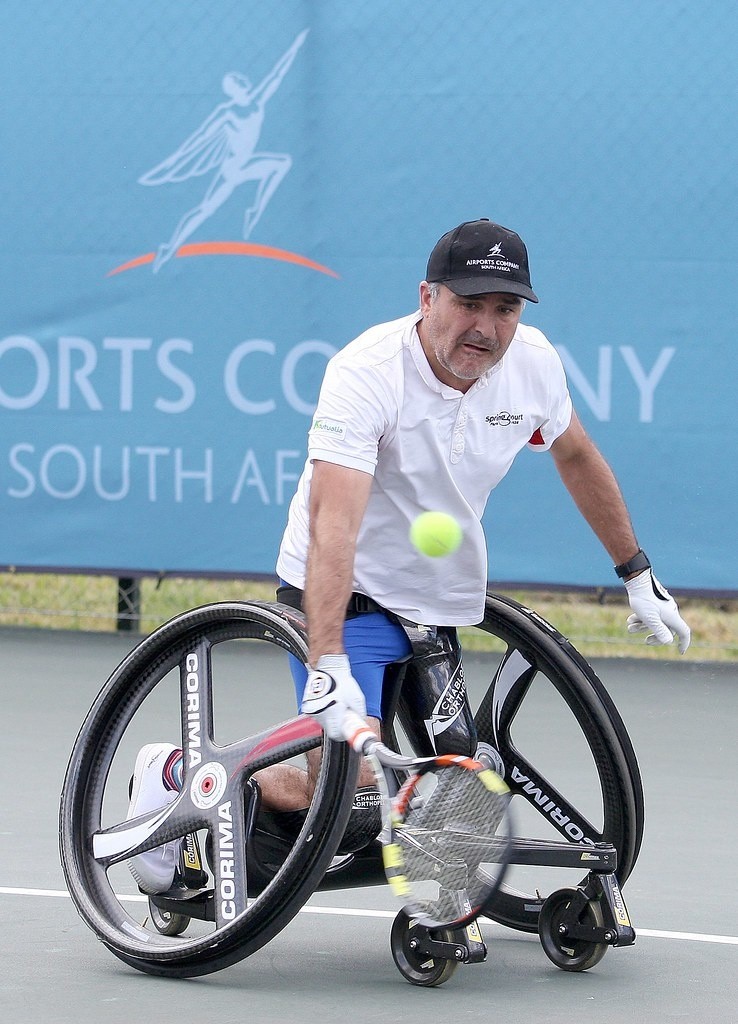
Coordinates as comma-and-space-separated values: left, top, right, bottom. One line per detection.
56, 589, 649, 990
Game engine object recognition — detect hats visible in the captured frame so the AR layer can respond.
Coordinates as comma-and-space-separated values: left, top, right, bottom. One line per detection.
425, 218, 538, 304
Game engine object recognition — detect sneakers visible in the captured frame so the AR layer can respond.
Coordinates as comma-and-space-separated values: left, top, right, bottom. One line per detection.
125, 741, 188, 895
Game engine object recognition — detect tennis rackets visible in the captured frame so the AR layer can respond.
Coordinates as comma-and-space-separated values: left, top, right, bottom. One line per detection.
346, 710, 515, 930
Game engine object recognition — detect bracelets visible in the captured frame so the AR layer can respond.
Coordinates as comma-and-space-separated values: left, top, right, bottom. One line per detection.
614, 547, 652, 579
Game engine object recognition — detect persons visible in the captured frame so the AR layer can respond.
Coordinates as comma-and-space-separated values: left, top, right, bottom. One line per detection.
126, 218, 690, 895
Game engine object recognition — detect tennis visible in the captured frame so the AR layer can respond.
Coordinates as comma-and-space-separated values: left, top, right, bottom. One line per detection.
411, 512, 462, 557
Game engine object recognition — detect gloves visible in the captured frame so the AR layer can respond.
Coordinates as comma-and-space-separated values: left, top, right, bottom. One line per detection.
301, 654, 368, 743
624, 566, 690, 653
